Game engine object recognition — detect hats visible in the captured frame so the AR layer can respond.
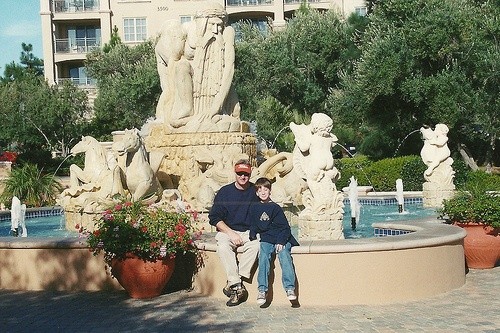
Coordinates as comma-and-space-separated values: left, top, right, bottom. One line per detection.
234, 164, 251, 174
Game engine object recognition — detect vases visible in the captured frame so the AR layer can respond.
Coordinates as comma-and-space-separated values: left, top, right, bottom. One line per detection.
109, 249, 176, 300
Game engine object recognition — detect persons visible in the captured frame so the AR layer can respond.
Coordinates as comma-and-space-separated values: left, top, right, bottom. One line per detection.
154, 0, 241, 127
300, 113, 338, 182
425, 124, 450, 175
249, 178, 297, 303
208, 158, 258, 306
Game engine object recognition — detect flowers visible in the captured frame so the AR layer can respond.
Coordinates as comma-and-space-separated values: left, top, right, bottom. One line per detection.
75, 190, 204, 279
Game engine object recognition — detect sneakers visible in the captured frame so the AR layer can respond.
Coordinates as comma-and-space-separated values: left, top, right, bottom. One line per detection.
286, 289, 297, 300
223, 280, 248, 306
256, 291, 268, 306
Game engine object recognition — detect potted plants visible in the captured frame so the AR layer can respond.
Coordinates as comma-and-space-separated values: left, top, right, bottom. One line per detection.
435, 186, 500, 269
